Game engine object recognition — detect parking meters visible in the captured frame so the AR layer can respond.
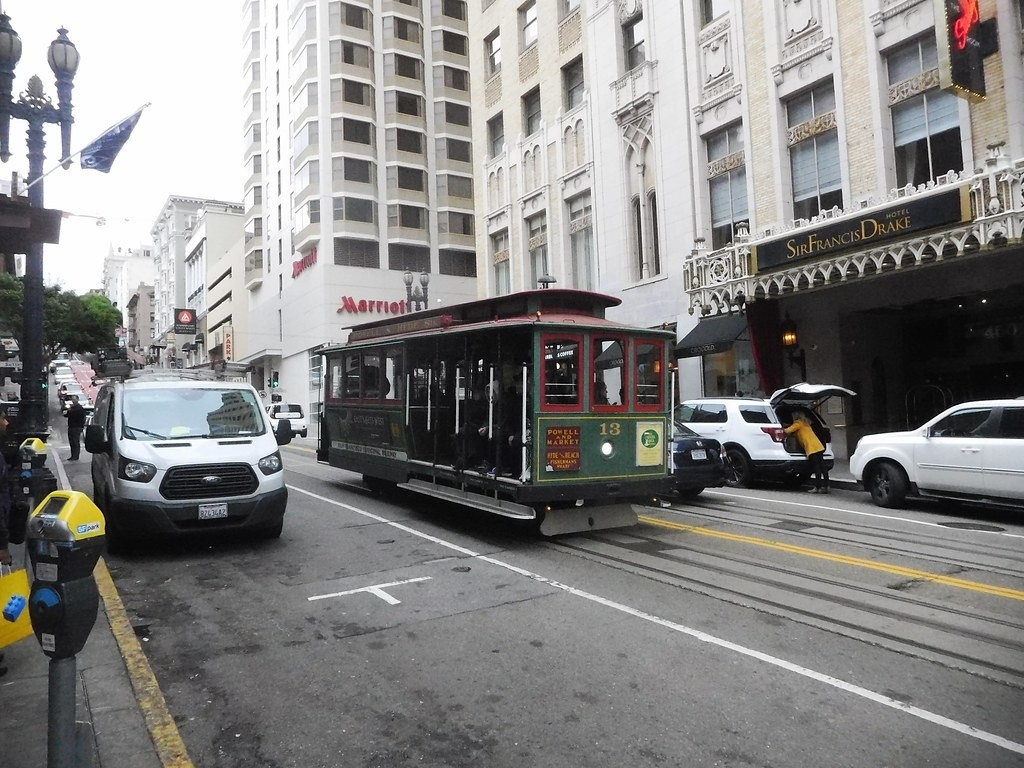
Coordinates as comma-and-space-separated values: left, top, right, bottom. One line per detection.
17, 435, 108, 767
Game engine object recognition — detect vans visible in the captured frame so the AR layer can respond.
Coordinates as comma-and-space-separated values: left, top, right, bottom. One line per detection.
82, 378, 293, 555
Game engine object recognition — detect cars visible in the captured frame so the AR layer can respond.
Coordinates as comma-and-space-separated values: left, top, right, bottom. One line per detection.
667, 417, 728, 502
263, 403, 307, 440
49, 351, 95, 414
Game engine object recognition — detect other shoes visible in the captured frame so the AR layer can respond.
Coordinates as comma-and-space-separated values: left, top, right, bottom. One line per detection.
818, 487, 828, 494
808, 486, 818, 493
67, 457, 78, 461
487, 469, 513, 477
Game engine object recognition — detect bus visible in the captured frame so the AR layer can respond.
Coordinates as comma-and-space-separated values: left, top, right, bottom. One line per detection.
312, 286, 679, 536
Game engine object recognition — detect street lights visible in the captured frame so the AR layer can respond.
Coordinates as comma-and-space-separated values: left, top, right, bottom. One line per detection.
0, 0, 79, 514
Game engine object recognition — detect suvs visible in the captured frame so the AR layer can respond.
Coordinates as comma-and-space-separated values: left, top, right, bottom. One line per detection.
668, 381, 858, 486
850, 395, 1023, 515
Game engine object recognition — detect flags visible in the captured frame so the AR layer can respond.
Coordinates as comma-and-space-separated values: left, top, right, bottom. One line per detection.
80, 107, 145, 173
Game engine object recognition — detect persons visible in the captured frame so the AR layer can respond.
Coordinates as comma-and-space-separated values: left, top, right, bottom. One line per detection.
337, 359, 390, 399
617, 374, 660, 404
410, 362, 617, 480
0, 392, 19, 676
149, 357, 153, 363
784, 411, 830, 493
63, 395, 86, 460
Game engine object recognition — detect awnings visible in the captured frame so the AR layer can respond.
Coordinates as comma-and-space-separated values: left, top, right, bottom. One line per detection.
594, 340, 654, 371
150, 343, 166, 348
194, 333, 204, 343
182, 342, 190, 352
673, 315, 751, 359
